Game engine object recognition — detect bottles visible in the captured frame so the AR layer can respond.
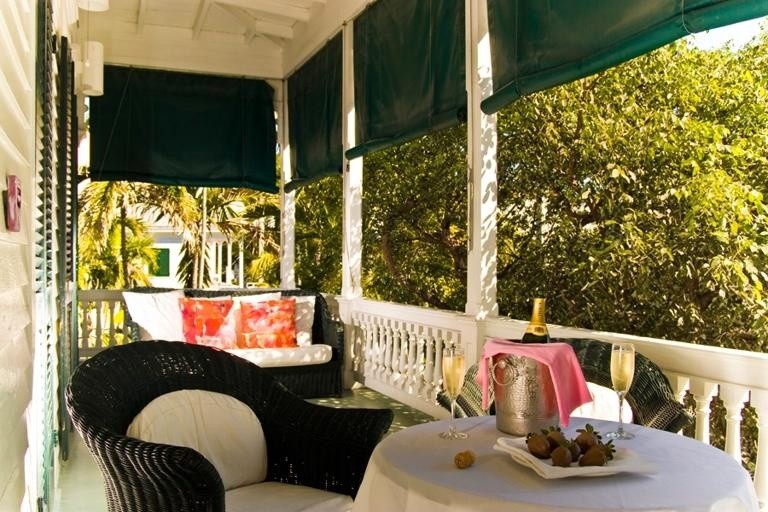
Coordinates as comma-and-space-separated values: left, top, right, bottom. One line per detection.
521, 295, 551, 343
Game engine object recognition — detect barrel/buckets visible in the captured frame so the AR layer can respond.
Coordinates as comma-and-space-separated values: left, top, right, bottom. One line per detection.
489, 340, 571, 437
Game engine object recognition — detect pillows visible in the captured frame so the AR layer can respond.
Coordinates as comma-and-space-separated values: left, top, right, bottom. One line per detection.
121, 289, 318, 350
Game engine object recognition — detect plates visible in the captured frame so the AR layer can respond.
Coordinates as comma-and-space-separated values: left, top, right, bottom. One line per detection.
510, 455, 620, 479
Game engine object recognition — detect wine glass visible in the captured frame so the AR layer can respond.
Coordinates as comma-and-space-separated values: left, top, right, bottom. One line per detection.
438, 347, 470, 441
604, 342, 637, 440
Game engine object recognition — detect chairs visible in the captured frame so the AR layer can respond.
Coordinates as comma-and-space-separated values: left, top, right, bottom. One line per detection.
64, 341, 397, 512
434, 335, 684, 428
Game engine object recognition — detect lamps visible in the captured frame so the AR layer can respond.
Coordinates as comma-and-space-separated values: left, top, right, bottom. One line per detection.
72, 40, 105, 97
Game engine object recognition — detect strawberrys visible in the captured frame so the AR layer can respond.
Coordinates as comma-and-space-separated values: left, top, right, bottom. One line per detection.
526, 424, 616, 466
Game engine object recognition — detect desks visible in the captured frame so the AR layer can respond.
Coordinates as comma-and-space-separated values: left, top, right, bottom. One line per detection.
367, 414, 751, 511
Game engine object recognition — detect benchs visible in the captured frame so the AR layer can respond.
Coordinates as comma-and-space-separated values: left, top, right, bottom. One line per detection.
120, 283, 343, 401
